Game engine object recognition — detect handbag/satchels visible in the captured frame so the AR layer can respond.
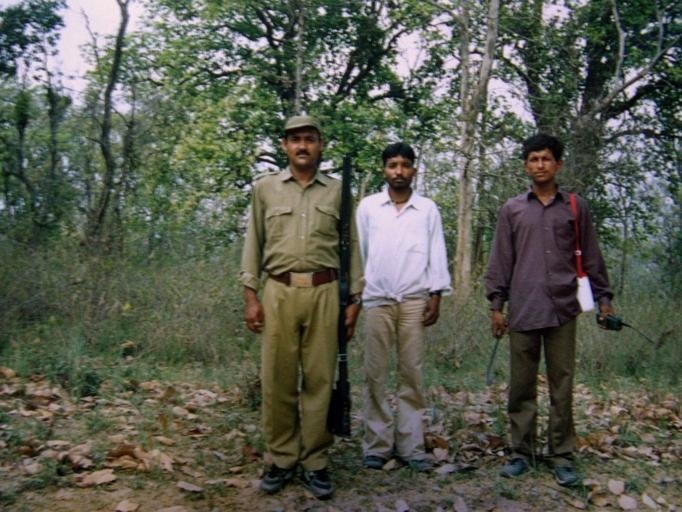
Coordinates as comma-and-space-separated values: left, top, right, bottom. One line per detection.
576, 271, 596, 314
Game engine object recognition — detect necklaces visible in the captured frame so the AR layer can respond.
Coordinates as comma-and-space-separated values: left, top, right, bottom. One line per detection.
391, 191, 412, 204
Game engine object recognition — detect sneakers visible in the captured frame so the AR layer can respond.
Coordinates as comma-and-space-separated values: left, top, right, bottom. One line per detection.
409, 459, 435, 473
260, 465, 296, 493
300, 464, 334, 500
547, 458, 579, 487
363, 455, 384, 469
499, 459, 537, 480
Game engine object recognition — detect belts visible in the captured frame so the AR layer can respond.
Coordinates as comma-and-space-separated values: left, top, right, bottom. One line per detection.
269, 268, 339, 287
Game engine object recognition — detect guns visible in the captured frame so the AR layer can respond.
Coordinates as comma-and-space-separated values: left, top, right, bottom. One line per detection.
327, 151, 355, 439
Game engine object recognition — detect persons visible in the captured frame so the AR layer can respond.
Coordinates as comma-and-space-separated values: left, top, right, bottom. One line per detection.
237, 115, 366, 501
353, 141, 454, 472
485, 133, 618, 488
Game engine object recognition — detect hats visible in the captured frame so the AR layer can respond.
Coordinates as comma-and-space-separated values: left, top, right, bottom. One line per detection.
284, 115, 322, 135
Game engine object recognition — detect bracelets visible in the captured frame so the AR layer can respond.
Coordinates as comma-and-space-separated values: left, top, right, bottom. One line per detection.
353, 297, 363, 306
429, 290, 442, 298
